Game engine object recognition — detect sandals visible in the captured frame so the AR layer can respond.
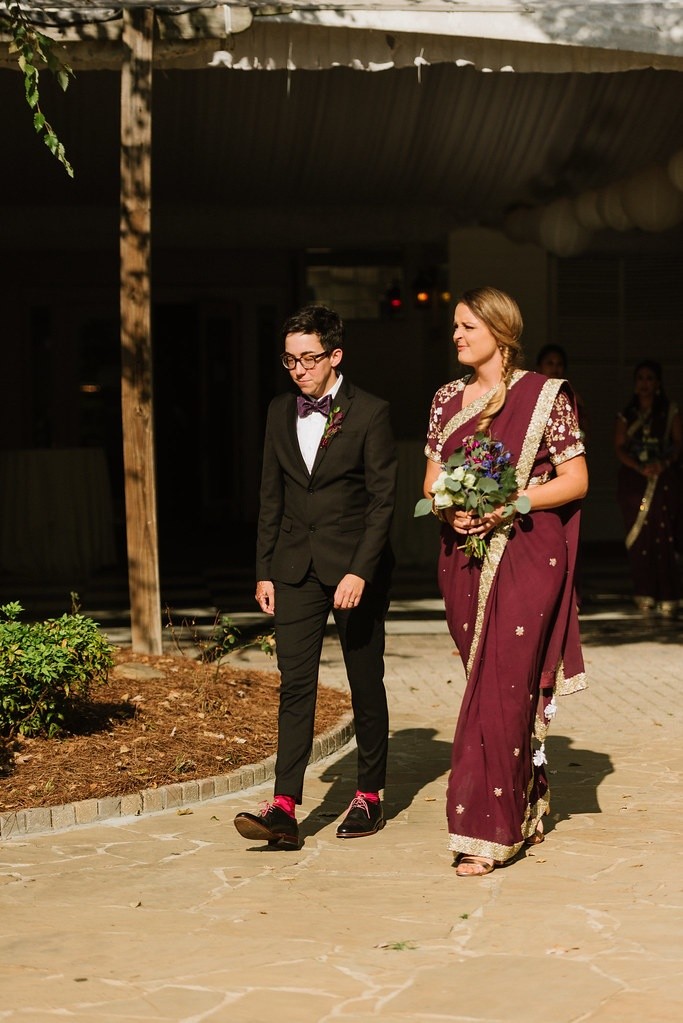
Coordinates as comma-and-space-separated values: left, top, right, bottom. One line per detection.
457, 853, 496, 877
528, 830, 545, 845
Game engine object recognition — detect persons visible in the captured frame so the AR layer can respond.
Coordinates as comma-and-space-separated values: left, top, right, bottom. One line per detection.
228, 303, 399, 852
612, 362, 682, 616
532, 343, 593, 617
422, 285, 589, 876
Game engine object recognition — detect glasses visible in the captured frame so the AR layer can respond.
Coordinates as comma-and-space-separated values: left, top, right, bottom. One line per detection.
280, 348, 332, 372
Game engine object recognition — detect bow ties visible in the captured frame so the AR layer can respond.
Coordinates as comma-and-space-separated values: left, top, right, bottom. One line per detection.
297, 393, 331, 417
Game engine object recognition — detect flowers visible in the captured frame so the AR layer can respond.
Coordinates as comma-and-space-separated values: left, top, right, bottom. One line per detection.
411, 430, 532, 563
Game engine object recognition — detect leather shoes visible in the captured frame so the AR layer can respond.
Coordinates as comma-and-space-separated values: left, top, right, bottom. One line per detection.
337, 798, 386, 839
232, 805, 298, 851
